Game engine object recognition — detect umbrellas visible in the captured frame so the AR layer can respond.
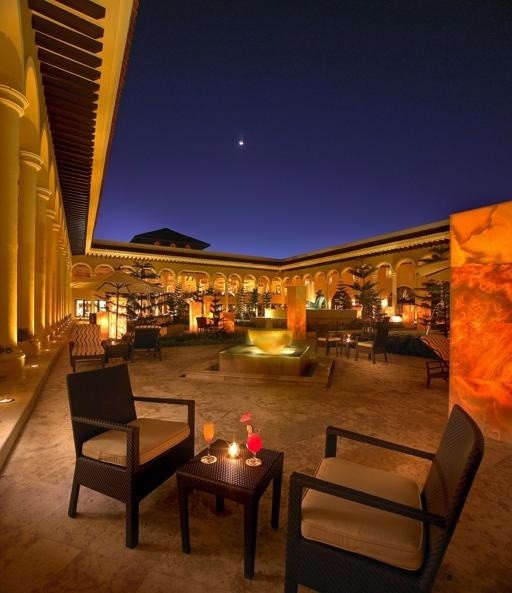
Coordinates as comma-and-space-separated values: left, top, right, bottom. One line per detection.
415, 260, 449, 285
70, 268, 165, 340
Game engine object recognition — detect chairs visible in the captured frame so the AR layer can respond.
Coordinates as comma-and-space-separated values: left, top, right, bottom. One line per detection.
418, 332, 450, 388
275, 404, 492, 593
59, 304, 209, 389
313, 320, 391, 364
61, 362, 202, 547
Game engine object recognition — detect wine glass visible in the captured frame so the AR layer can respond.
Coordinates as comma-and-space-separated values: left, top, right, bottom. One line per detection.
245, 432, 263, 467
201, 422, 217, 464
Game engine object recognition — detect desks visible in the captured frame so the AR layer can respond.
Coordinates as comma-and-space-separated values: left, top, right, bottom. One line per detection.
176, 435, 287, 578
